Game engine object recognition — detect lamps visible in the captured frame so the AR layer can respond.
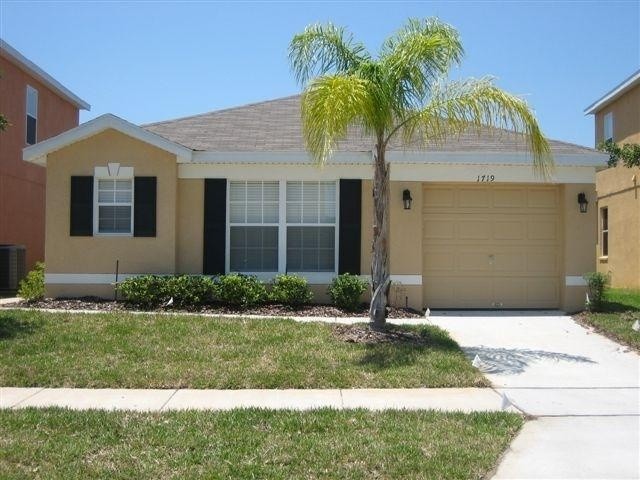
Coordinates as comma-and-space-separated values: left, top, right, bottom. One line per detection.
402, 186, 414, 209
576, 192, 588, 215
